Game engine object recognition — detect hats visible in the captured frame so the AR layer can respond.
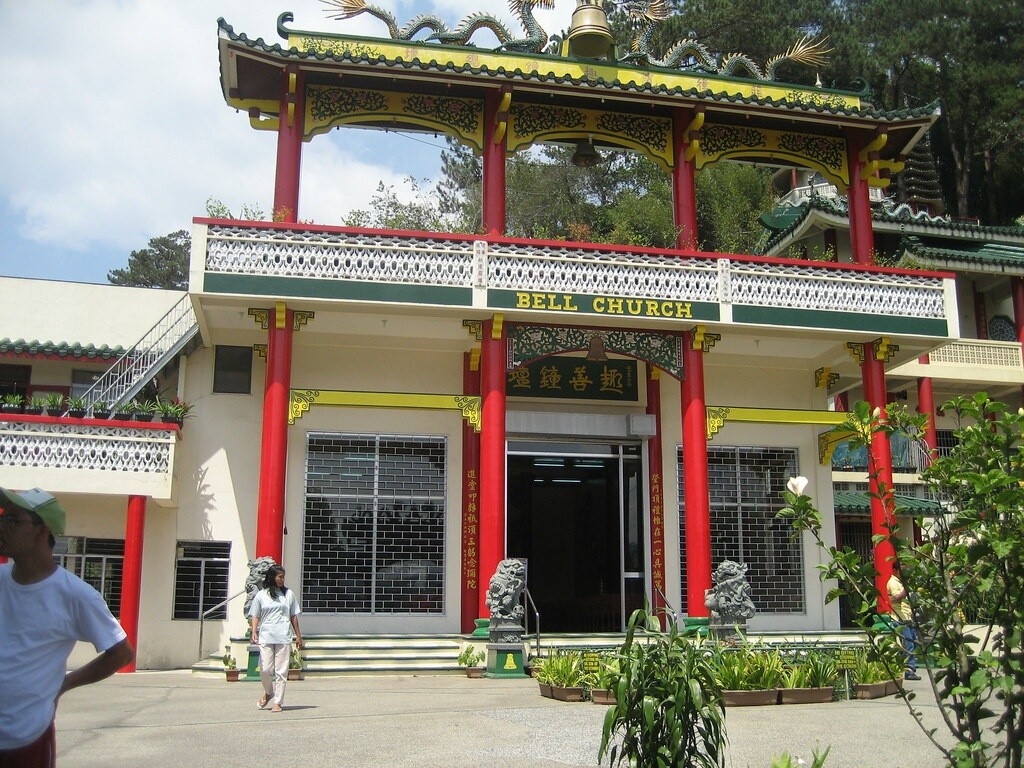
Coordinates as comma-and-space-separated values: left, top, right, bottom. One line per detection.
0, 487, 67, 539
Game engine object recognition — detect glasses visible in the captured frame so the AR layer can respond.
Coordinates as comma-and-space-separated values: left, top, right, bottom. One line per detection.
1, 513, 40, 528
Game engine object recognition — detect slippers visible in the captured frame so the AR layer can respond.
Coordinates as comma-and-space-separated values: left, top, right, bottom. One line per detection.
257, 692, 275, 709
272, 704, 282, 712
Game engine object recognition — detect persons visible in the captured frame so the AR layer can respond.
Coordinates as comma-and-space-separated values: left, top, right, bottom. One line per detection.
247, 564, 302, 712
885, 561, 921, 680
0, 486, 134, 768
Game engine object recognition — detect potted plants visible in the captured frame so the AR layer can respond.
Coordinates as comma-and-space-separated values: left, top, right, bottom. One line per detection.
222, 655, 241, 681
525, 621, 905, 705
288, 649, 303, 679
0, 384, 199, 430
458, 644, 486, 678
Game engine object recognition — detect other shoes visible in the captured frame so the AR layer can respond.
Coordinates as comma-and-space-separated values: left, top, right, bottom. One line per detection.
905, 672, 922, 680
